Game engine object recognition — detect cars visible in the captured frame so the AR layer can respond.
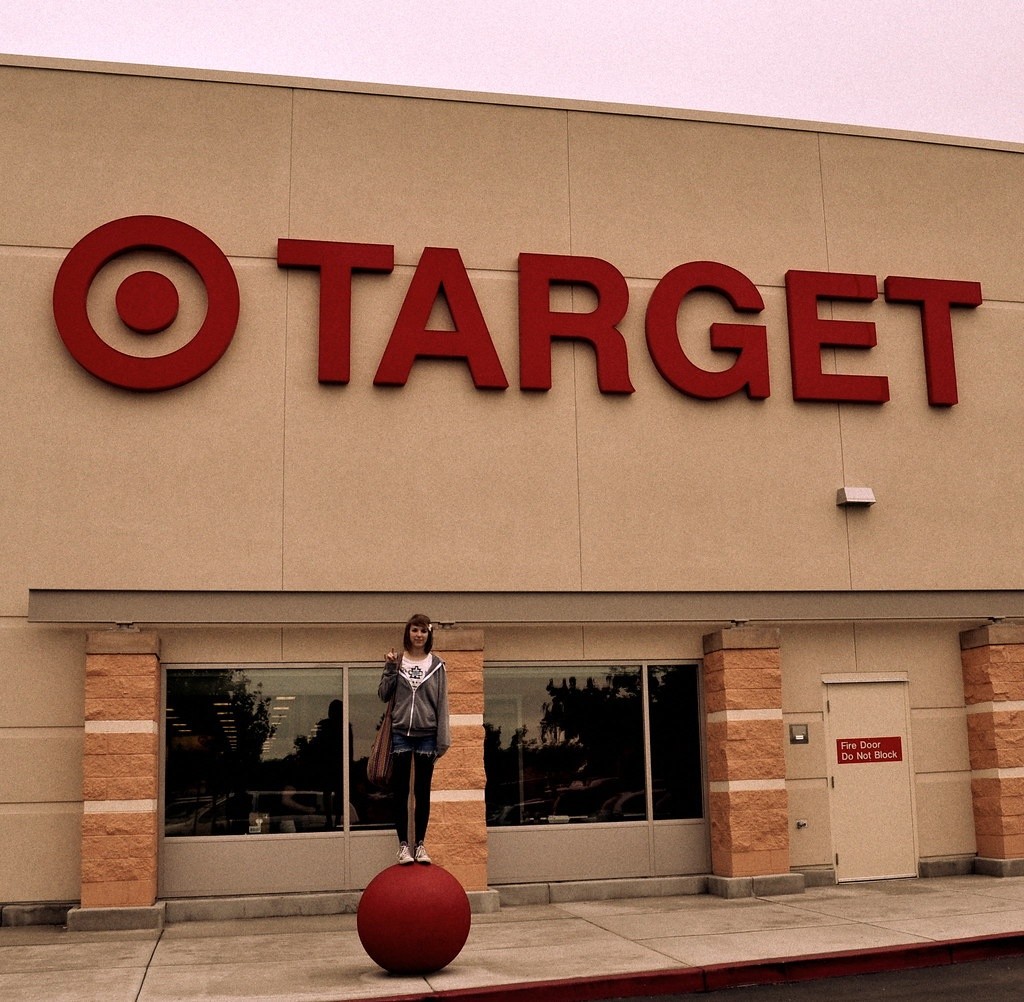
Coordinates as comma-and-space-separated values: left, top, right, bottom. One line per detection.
164, 776, 685, 836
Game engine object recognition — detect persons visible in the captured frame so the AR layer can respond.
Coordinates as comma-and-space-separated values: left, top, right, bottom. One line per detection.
316, 700, 354, 829
378, 613, 451, 864
280, 780, 321, 816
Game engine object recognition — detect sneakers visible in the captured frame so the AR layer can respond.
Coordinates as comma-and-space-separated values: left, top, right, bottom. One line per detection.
396, 845, 414, 864
413, 844, 431, 863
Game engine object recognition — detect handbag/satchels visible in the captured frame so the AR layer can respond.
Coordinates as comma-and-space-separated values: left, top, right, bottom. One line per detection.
367, 716, 392, 785
